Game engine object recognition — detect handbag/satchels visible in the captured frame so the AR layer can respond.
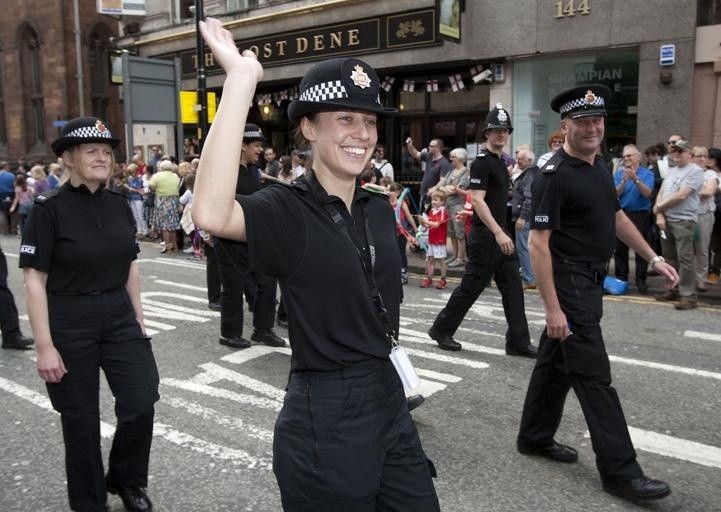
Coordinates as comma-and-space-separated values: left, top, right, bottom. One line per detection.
389, 344, 421, 392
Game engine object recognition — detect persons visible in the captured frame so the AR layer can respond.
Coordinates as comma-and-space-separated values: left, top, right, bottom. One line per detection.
192, 14, 444, 511
501, 131, 565, 290
194, 146, 314, 329
106, 135, 204, 260
1, 250, 35, 349
220, 122, 288, 347
358, 137, 475, 288
1, 157, 71, 238
19, 115, 162, 511
518, 83, 681, 500
610, 134, 721, 309
429, 104, 541, 358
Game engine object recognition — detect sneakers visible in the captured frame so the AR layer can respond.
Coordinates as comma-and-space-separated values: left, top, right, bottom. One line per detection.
636, 272, 721, 310
523, 283, 536, 289
401, 257, 466, 289
160, 240, 203, 256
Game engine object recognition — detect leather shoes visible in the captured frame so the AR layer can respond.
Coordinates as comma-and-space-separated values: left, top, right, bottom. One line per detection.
519, 436, 577, 461
604, 476, 670, 499
209, 300, 289, 348
2, 332, 34, 349
406, 394, 424, 412
105, 475, 152, 512
507, 343, 538, 358
428, 325, 461, 350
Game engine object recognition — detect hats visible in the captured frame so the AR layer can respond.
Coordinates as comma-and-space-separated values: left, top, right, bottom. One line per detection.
672, 139, 692, 150
551, 83, 612, 119
484, 105, 513, 134
52, 116, 123, 155
287, 58, 399, 123
243, 123, 267, 141
